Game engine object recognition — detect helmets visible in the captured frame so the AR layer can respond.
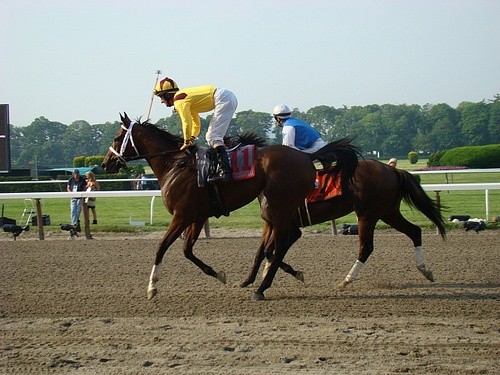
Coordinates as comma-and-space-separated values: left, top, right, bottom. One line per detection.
270, 104, 292, 119
152, 77, 180, 94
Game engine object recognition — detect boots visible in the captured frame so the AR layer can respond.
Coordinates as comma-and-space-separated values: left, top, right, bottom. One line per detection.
208, 144, 232, 182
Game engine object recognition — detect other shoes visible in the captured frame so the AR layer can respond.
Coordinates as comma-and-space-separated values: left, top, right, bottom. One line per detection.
91, 220, 98, 224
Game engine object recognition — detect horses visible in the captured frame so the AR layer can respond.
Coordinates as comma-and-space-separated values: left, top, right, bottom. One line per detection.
103, 111, 367, 301
223, 131, 449, 289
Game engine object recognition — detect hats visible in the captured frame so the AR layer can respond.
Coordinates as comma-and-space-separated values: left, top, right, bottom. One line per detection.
73, 169, 79, 174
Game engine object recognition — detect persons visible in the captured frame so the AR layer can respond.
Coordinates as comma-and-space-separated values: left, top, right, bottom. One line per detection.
82, 171, 99, 224
389, 158, 396, 167
152, 77, 238, 183
273, 104, 328, 189
67, 169, 88, 225
